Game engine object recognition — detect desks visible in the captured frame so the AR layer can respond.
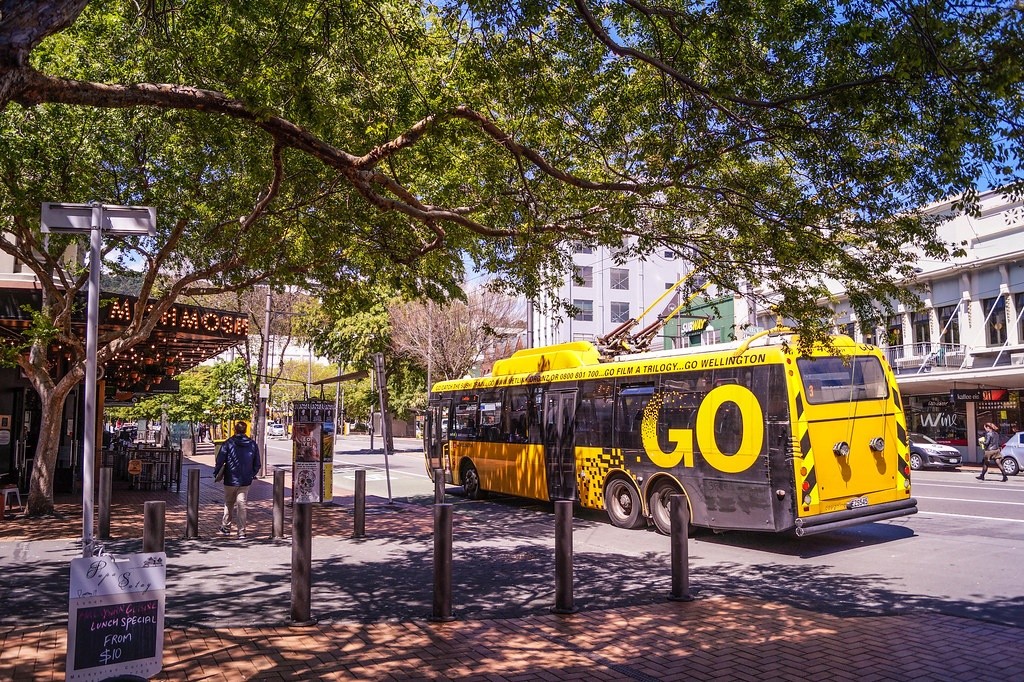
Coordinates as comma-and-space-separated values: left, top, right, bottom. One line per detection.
115, 440, 183, 489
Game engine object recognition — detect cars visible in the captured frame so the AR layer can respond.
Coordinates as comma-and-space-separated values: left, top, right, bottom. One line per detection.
999, 431, 1024, 476
908, 431, 963, 472
267, 420, 285, 437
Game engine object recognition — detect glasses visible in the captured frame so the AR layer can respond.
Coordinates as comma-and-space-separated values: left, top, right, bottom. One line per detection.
232, 427, 235, 431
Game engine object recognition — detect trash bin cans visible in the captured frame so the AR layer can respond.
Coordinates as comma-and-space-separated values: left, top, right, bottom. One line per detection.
212, 440, 227, 463
344, 423, 350, 436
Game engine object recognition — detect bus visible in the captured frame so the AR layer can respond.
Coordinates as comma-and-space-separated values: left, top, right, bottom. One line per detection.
421, 326, 918, 538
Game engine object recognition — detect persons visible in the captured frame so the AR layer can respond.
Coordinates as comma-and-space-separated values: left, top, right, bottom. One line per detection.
102, 425, 111, 450
452, 419, 478, 437
196, 425, 204, 443
213, 422, 261, 539
120, 428, 129, 445
130, 427, 136, 442
975, 423, 1008, 482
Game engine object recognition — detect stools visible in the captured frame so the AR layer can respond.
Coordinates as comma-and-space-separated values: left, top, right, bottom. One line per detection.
137, 462, 154, 490
151, 460, 170, 490
0, 487, 23, 514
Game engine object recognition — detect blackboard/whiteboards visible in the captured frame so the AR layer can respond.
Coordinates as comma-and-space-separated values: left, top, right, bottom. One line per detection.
65, 590, 165, 682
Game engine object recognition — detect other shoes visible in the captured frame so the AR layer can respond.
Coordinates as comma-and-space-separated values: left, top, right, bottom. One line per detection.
218, 522, 231, 535
237, 528, 247, 540
975, 473, 984, 480
1001, 476, 1008, 482
